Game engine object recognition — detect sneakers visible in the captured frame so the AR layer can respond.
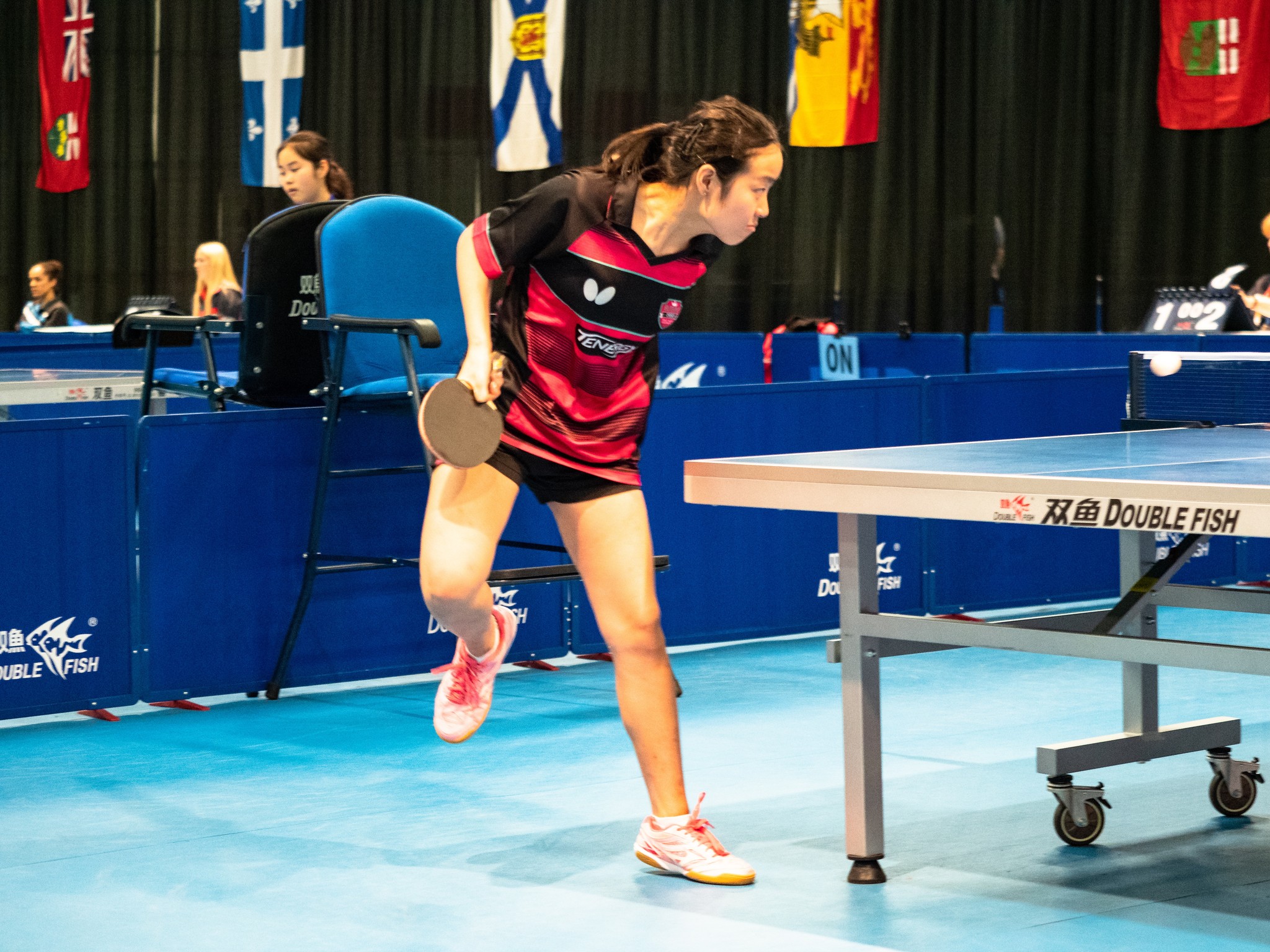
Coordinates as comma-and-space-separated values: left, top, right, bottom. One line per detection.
634, 792, 756, 886
431, 605, 516, 743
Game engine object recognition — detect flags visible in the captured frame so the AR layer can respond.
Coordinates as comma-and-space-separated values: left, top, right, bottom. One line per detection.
490, 0, 566, 172
1156, 0, 1270, 131
788, 0, 881, 147
35, 0, 93, 193
239, 0, 305, 188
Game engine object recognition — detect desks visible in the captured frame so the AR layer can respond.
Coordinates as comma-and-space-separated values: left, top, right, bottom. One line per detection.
681, 348, 1270, 885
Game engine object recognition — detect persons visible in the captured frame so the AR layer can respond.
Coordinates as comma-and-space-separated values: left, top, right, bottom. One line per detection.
1229, 214, 1270, 330
275, 130, 354, 205
193, 242, 242, 319
420, 95, 783, 886
15, 261, 68, 332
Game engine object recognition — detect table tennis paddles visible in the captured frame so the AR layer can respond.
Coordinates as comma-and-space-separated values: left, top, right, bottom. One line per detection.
418, 351, 509, 469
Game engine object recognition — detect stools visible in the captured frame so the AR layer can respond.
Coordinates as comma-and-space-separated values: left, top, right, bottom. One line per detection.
123, 197, 357, 503
262, 194, 685, 700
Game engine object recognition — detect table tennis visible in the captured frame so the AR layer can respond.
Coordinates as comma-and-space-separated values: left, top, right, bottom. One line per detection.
1149, 352, 1183, 377
